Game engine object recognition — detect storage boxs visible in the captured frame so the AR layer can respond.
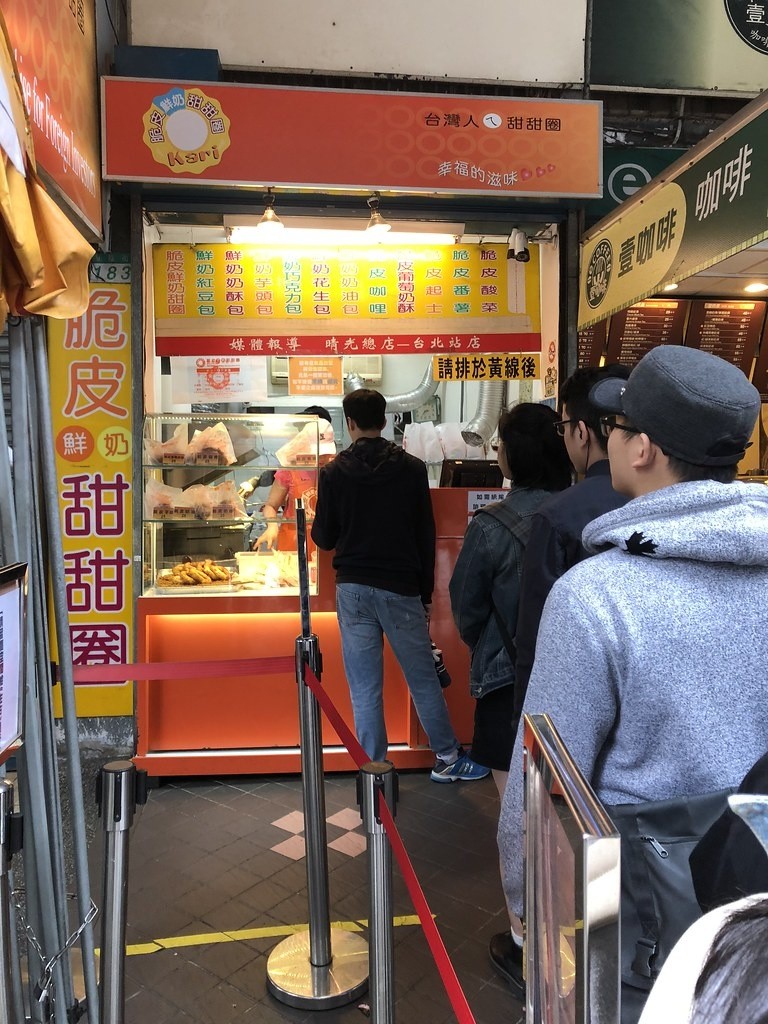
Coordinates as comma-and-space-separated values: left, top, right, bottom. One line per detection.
152, 507, 174, 520
174, 506, 195, 520
211, 505, 236, 520
235, 550, 299, 576
196, 452, 222, 466
163, 453, 186, 466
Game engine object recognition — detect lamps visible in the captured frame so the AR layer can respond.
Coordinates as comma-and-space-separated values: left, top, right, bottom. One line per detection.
258, 193, 284, 231
364, 197, 392, 234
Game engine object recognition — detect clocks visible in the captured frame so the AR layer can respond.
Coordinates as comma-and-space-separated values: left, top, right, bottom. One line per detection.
413, 396, 438, 423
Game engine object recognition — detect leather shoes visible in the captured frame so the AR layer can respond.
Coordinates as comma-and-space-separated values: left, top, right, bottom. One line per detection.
489, 930, 527, 1004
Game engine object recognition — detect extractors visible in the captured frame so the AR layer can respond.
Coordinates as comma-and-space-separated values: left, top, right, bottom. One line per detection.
161, 404, 259, 489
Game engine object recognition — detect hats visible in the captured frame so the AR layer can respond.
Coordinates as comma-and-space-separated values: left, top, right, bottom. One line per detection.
589, 345, 762, 468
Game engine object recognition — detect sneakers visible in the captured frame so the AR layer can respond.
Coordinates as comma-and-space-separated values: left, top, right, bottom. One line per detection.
431, 748, 491, 783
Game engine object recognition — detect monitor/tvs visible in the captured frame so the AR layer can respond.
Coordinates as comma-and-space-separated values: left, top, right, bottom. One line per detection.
440, 459, 504, 490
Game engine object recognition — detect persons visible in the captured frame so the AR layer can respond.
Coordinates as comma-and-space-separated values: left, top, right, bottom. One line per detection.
489, 363, 636, 731
238, 406, 337, 553
496, 346, 767, 1024
311, 390, 491, 782
449, 400, 572, 1001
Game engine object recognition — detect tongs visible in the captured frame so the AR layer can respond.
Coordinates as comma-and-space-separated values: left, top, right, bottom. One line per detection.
256, 543, 277, 556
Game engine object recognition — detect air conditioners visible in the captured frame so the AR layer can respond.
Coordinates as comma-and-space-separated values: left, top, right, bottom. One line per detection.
270, 355, 383, 387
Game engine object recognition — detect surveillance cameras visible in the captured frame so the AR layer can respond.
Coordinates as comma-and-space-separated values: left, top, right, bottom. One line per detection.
506, 228, 530, 263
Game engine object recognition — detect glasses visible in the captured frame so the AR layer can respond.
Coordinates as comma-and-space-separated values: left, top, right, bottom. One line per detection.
598, 415, 641, 437
554, 417, 580, 436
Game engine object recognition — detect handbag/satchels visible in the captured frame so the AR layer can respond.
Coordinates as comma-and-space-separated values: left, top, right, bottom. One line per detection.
592, 785, 738, 1024
